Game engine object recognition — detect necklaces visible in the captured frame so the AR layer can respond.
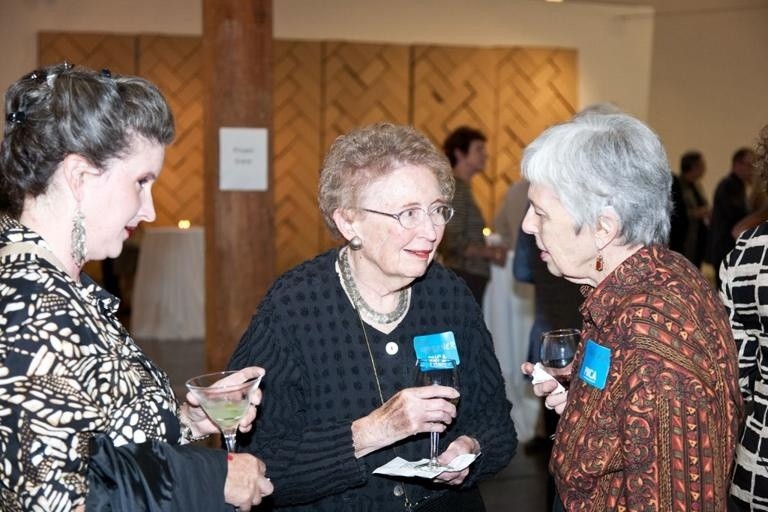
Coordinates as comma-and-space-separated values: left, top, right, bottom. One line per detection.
333, 244, 410, 324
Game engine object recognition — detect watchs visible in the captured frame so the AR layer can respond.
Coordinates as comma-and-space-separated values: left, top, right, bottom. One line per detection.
471, 437, 481, 459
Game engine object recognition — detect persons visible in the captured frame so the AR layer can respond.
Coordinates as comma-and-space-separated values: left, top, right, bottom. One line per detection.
433, 125, 509, 312
667, 146, 758, 294
715, 120, 768, 512
519, 98, 747, 512
1, 59, 275, 512
513, 228, 592, 459
218, 120, 518, 512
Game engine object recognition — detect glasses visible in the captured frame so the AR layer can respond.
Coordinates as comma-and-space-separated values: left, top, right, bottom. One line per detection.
354, 204, 456, 229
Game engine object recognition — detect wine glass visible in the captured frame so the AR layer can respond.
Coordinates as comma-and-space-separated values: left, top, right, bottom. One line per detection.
185, 370, 267, 452
414, 357, 462, 474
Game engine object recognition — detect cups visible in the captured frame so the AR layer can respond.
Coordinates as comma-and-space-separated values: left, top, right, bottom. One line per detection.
538, 328, 588, 393
